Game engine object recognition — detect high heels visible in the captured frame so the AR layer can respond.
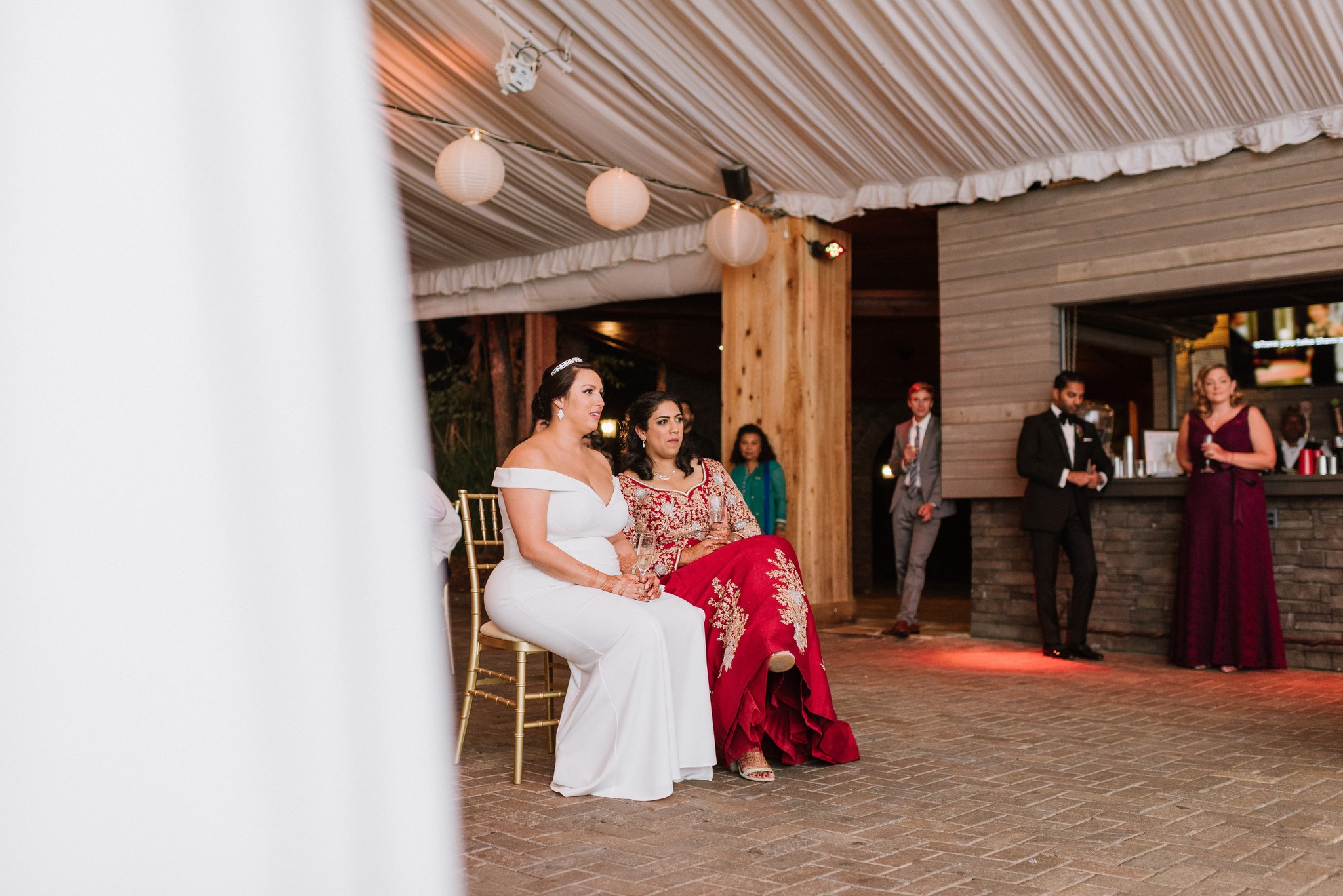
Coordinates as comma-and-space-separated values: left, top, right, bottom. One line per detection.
768, 652, 795, 673
730, 760, 776, 781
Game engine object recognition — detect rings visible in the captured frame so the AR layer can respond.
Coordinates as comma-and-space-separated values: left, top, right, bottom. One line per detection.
715, 537, 718, 539
659, 588, 662, 591
1210, 450, 1213, 456
637, 588, 643, 594
655, 585, 659, 590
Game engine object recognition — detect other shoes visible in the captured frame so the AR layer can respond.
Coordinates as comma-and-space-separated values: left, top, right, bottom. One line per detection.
1196, 665, 1208, 670
1221, 666, 1238, 673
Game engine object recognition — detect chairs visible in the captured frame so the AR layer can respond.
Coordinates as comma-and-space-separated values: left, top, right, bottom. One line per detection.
445, 489, 569, 785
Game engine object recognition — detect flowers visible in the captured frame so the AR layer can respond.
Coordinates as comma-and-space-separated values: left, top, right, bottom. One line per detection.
1075, 423, 1083, 441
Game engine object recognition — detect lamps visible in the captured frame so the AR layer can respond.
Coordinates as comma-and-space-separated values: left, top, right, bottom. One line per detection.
806, 238, 844, 262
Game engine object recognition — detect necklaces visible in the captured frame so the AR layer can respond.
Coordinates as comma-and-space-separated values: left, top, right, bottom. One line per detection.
652, 469, 677, 480
1211, 420, 1217, 426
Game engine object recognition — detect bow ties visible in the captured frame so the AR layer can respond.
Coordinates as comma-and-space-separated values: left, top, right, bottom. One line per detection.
1058, 411, 1077, 425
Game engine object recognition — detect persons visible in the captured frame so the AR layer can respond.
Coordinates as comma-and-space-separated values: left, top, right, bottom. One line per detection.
1305, 303, 1343, 384
1169, 363, 1287, 673
674, 395, 716, 461
482, 355, 717, 802
728, 423, 788, 539
614, 391, 861, 783
1224, 311, 1258, 388
881, 383, 957, 638
1015, 370, 1115, 661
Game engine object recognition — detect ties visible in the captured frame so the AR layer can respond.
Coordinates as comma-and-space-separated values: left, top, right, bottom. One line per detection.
908, 425, 920, 499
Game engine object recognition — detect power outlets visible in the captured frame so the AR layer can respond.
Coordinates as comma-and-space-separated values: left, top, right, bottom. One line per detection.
1268, 506, 1278, 530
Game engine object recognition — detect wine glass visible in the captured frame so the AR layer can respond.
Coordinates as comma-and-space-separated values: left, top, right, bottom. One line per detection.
909, 439, 919, 467
1201, 433, 1215, 473
636, 533, 657, 576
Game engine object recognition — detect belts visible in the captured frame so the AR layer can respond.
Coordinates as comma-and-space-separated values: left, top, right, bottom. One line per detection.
902, 486, 922, 494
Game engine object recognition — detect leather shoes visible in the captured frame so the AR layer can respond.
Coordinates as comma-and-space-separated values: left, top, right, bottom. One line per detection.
894, 620, 909, 639
1069, 645, 1105, 661
1043, 647, 1073, 660
882, 621, 920, 636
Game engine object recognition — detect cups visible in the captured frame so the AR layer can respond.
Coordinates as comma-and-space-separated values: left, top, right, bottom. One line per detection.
1298, 449, 1338, 475
709, 496, 724, 524
1113, 435, 1144, 479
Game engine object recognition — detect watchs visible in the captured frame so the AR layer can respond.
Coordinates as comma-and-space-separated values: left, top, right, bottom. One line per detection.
733, 534, 738, 542
